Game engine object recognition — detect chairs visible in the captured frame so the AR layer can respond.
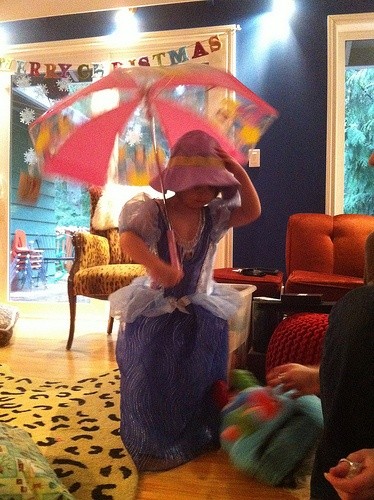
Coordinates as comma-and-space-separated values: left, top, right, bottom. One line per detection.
10, 230, 75, 292
66, 227, 147, 349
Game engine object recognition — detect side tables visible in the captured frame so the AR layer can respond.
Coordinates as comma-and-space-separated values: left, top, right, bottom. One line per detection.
212, 266, 283, 299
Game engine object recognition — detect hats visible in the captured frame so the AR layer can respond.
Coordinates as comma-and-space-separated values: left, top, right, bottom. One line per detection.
149, 128, 239, 201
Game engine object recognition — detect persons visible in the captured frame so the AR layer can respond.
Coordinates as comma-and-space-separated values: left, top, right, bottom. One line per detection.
107, 130, 262, 471
267, 231, 373, 500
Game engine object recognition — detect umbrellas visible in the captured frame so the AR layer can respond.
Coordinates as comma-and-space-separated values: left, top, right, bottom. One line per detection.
27, 64, 280, 289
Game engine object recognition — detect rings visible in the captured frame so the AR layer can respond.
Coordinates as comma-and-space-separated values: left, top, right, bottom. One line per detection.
277, 373, 289, 388
337, 458, 358, 476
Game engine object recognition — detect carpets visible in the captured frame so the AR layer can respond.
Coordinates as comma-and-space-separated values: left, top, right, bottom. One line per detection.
0, 362, 139, 500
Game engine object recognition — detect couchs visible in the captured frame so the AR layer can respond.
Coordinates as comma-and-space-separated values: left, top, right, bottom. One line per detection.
285, 213, 374, 281
